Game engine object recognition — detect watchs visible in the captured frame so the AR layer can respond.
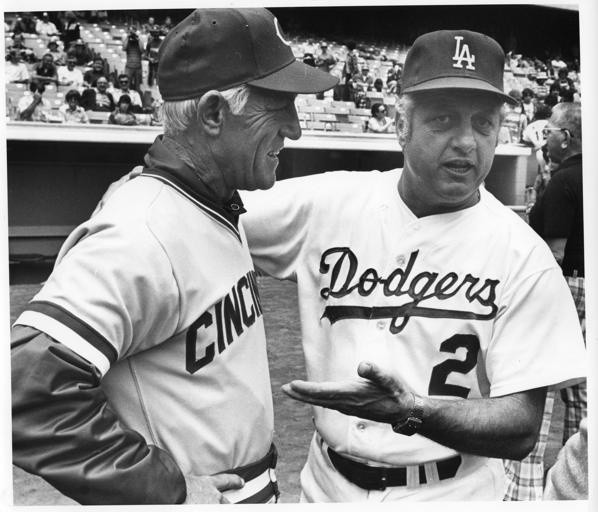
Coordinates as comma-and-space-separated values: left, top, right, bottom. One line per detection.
394, 394, 424, 438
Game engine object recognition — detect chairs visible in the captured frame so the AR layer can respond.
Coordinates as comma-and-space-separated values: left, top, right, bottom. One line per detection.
7, 23, 415, 134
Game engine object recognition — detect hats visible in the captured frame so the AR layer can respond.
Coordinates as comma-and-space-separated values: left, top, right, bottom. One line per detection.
157, 9, 340, 102
401, 29, 518, 102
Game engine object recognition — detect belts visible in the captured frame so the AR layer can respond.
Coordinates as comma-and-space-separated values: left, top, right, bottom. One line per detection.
320, 440, 461, 491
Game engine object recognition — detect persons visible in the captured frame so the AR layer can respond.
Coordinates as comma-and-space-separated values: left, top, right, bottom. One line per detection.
498, 100, 589, 504
9, 7, 339, 503
5, 11, 580, 144
90, 30, 589, 502
